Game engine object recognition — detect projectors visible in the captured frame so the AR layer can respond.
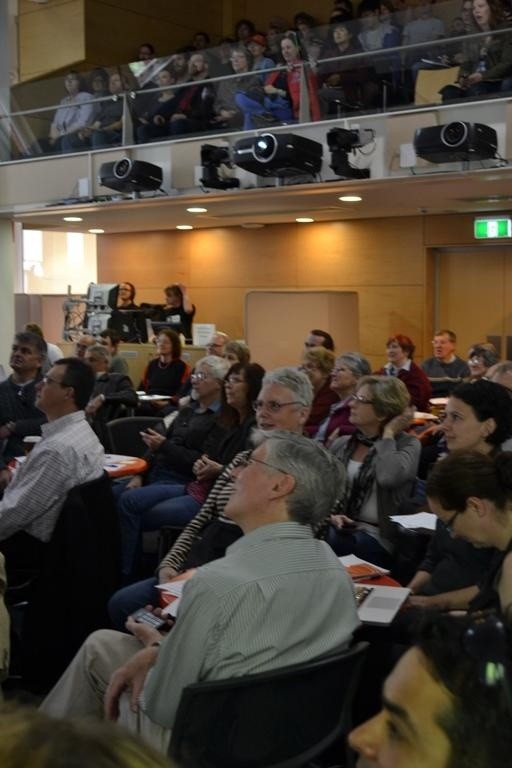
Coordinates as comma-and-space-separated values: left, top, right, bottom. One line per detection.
98, 158, 162, 193
234, 133, 322, 177
413, 120, 497, 163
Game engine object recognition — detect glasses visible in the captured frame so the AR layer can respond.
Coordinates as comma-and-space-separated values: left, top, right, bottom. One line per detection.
43, 375, 76, 393
252, 399, 306, 415
238, 449, 289, 477
351, 392, 373, 405
442, 607, 507, 749
436, 505, 469, 534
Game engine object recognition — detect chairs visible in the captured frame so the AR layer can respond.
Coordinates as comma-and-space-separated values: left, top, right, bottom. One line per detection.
1, 374, 512, 767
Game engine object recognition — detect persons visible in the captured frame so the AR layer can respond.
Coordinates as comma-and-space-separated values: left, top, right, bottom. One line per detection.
439, 3, 509, 103
215, 50, 267, 132
124, 67, 182, 146
245, 36, 276, 83
265, 4, 354, 70
46, 70, 98, 154
78, 74, 148, 150
353, 2, 403, 99
85, 68, 113, 114
233, 20, 257, 50
1, 325, 512, 768
108, 285, 149, 345
401, 2, 446, 80
157, 286, 193, 344
164, 48, 194, 99
237, 33, 319, 131
183, 31, 211, 60
129, 44, 158, 89
215, 39, 239, 75
317, 21, 367, 120
147, 53, 220, 140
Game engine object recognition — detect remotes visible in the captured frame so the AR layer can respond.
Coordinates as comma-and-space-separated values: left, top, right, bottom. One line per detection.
132, 608, 165, 629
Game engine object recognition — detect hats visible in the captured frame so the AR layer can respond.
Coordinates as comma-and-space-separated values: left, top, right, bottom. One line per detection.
247, 34, 268, 48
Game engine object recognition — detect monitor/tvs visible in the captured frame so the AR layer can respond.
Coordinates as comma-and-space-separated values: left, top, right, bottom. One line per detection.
151, 322, 184, 336
82, 313, 119, 334
86, 283, 119, 313
118, 310, 148, 344
